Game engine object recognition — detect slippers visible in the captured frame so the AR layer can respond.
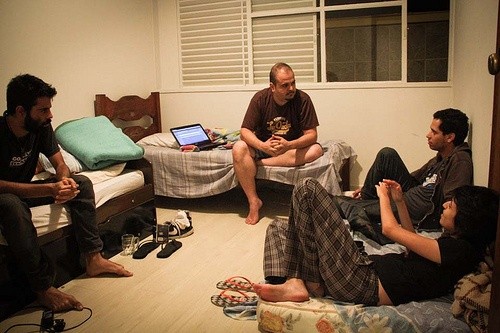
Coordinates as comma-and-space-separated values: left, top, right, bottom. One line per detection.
157, 238, 183, 258
133, 240, 161, 258
217, 276, 257, 292
211, 288, 259, 307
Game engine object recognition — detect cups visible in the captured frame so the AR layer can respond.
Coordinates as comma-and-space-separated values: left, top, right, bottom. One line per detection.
121, 234, 139, 255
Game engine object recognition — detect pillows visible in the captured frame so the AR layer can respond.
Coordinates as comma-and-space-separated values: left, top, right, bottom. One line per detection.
135, 132, 180, 149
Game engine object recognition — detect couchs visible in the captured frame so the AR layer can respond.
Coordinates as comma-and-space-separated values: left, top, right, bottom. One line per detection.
255, 190, 493, 333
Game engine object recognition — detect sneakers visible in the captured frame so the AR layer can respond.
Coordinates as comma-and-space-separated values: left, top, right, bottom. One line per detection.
152, 223, 193, 241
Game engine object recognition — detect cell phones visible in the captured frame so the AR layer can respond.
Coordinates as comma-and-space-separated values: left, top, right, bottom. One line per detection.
42, 310, 53, 329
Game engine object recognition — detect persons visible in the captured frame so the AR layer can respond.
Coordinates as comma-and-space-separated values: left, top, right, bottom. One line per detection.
333, 108, 473, 232
233, 63, 323, 225
253, 177, 499, 306
0, 74, 133, 311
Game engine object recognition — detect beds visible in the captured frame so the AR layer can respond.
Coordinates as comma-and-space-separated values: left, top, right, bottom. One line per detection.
0, 114, 157, 323
93, 91, 358, 199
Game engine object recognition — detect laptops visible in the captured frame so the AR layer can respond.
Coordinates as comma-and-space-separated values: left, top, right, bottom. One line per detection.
170, 123, 227, 150
